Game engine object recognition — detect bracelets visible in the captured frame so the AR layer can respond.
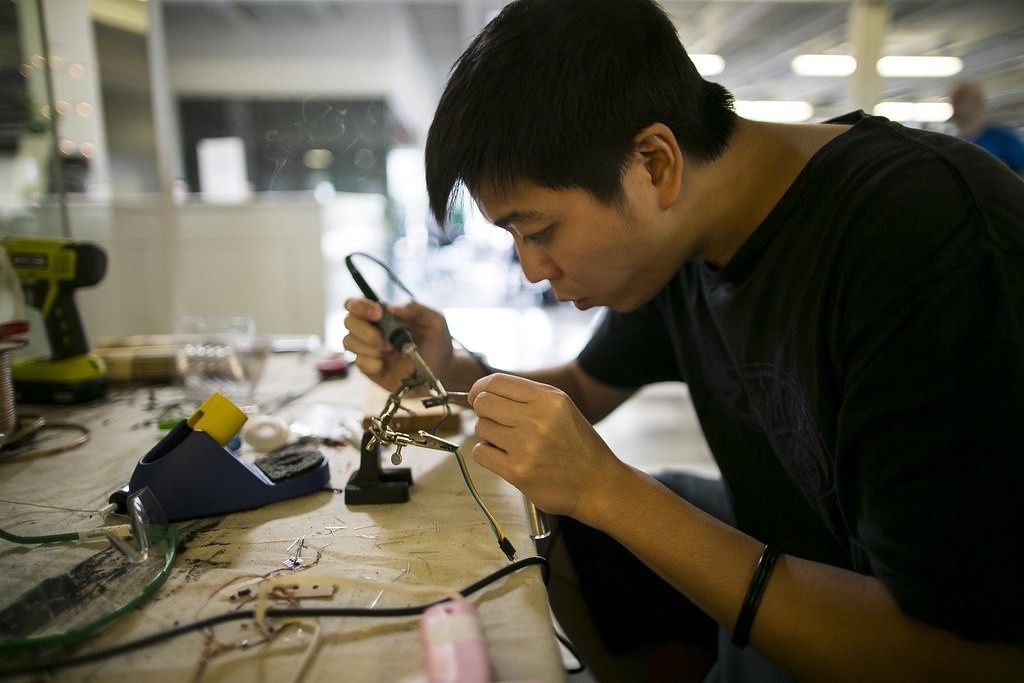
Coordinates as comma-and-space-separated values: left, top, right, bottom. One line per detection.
731, 543, 782, 651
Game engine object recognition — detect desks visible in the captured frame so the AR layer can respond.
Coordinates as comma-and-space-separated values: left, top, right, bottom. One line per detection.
0, 338, 564, 683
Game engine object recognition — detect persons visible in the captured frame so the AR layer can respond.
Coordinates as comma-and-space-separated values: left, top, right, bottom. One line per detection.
949, 84, 1024, 175
343, 2, 1024, 683
